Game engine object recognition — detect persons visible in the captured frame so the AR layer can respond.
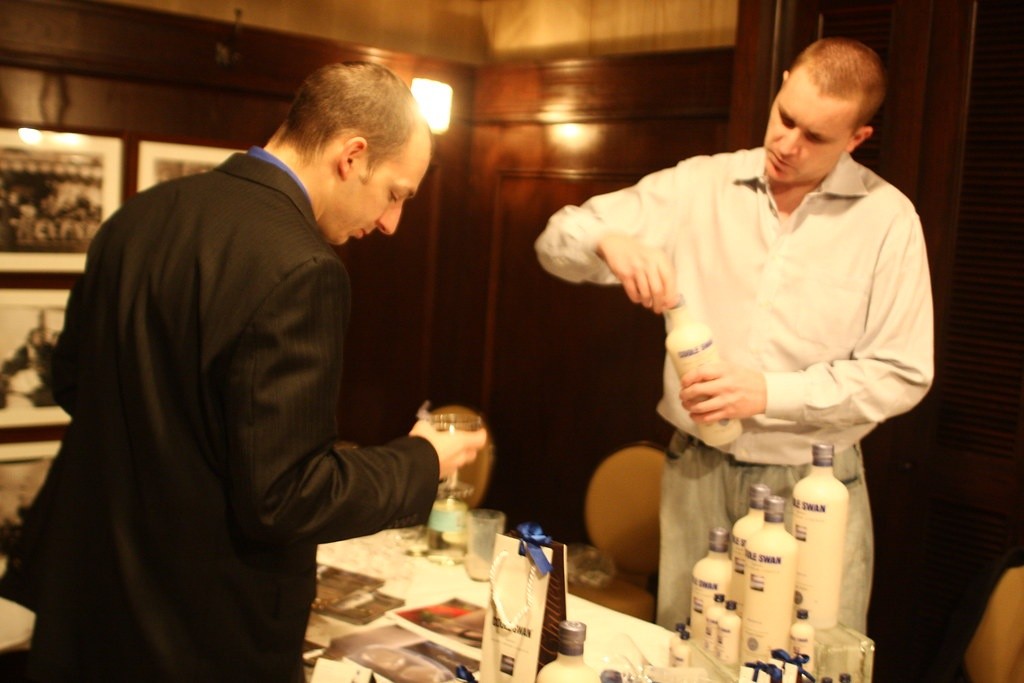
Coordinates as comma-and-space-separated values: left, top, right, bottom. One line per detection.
533, 35, 935, 639
0, 61, 489, 683
2, 329, 62, 406
0, 151, 106, 252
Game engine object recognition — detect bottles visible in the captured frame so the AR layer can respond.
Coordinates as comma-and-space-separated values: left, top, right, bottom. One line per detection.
789, 609, 815, 675
705, 593, 741, 664
665, 294, 744, 448
793, 442, 849, 628
691, 527, 735, 640
538, 620, 602, 683
732, 483, 799, 666
669, 616, 690, 668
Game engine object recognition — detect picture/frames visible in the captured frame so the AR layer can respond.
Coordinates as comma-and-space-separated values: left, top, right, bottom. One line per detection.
0, 119, 266, 445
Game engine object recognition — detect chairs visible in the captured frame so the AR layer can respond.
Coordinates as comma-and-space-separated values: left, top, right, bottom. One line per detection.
958, 565, 1023, 683
418, 404, 496, 508
567, 440, 670, 622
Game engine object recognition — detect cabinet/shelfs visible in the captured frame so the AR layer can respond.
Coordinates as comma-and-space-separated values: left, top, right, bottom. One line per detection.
718, 3, 1024, 681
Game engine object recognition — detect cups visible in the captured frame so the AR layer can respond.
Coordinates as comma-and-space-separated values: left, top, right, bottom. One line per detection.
464, 508, 505, 582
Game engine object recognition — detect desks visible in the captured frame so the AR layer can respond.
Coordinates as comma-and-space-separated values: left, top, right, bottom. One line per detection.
303, 525, 676, 683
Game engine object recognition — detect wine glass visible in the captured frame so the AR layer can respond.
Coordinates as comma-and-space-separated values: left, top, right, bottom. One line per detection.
427, 414, 480, 497
